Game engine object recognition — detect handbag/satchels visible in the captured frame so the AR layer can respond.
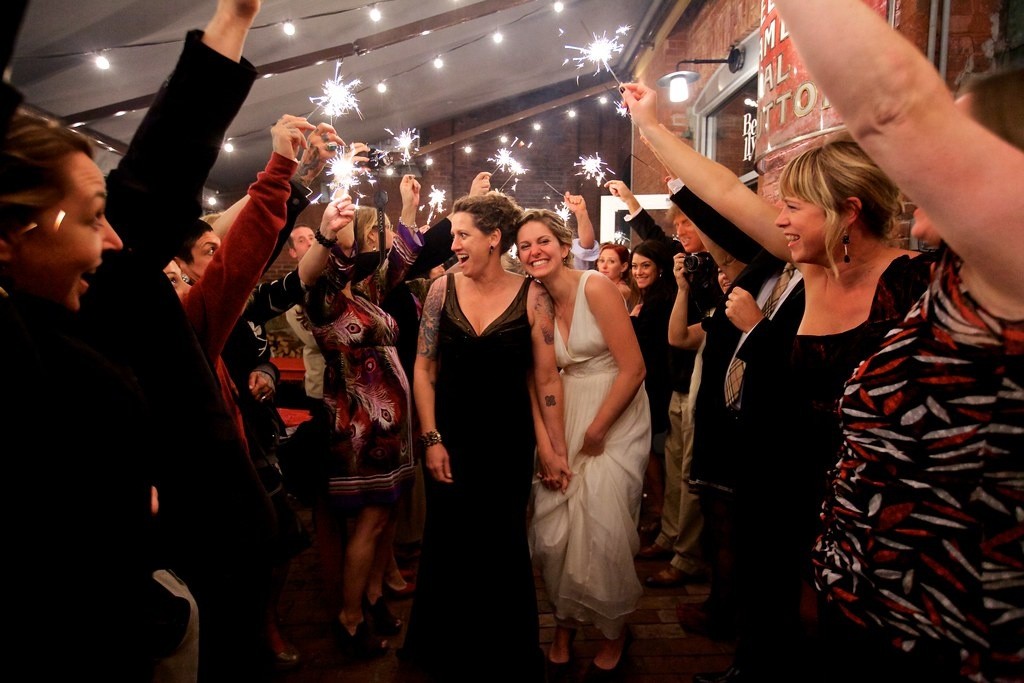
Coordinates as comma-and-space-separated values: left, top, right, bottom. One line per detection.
257, 464, 312, 563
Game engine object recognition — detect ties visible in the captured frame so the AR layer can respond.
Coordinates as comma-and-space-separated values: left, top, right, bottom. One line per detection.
724, 262, 797, 406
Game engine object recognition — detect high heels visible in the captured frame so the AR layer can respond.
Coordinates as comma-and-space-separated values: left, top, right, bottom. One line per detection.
334, 617, 388, 656
362, 591, 402, 634
586, 623, 634, 681
547, 627, 578, 678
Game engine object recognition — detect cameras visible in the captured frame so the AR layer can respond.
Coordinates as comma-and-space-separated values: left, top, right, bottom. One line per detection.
365, 146, 393, 171
682, 251, 714, 275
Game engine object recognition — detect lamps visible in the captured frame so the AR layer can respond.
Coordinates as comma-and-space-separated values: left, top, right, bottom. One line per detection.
657, 42, 744, 103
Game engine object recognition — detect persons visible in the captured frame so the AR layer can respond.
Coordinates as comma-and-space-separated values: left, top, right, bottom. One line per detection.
0, 0, 1024, 683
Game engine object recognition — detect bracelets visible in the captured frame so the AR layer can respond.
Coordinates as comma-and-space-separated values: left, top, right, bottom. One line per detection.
421, 430, 442, 447
313, 226, 338, 249
399, 216, 418, 232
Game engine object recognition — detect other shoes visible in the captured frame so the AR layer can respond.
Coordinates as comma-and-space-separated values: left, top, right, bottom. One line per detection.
273, 643, 301, 670
383, 579, 416, 597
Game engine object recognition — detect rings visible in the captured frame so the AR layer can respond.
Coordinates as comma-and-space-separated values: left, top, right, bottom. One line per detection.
262, 396, 266, 401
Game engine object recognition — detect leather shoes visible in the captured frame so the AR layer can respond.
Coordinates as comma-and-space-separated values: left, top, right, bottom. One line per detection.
636, 542, 672, 560
646, 566, 675, 586
694, 665, 745, 683
